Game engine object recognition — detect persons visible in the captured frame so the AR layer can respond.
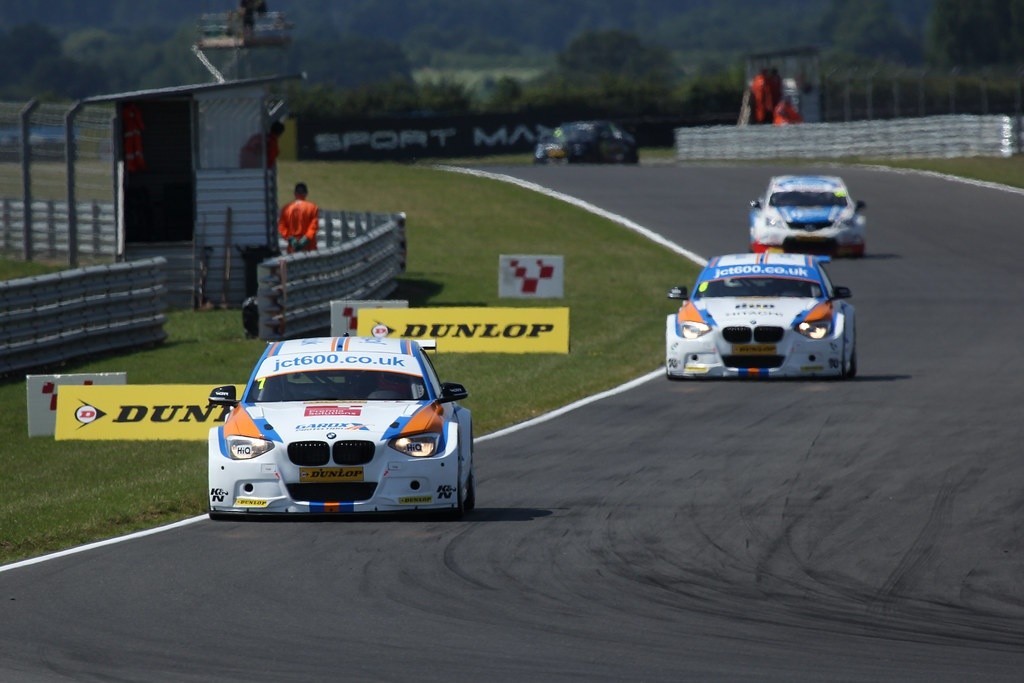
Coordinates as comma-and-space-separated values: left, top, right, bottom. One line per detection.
239, 120, 285, 168
278, 182, 320, 254
751, 65, 804, 125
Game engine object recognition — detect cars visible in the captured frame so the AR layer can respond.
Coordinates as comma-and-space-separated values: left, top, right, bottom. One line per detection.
749, 173, 868, 260
664, 249, 856, 384
203, 336, 476, 524
536, 123, 641, 167
